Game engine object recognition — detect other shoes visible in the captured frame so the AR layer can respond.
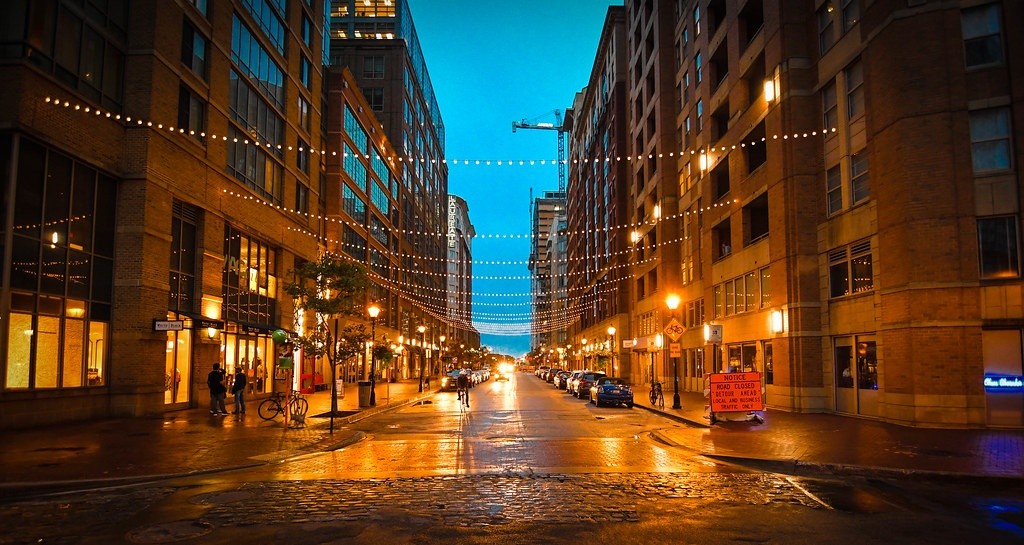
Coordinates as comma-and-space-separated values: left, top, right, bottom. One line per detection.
240, 411, 245, 413
222, 413, 230, 415
467, 404, 469, 407
232, 412, 239, 414
458, 398, 461, 400
213, 413, 217, 416
210, 409, 214, 414
217, 409, 222, 412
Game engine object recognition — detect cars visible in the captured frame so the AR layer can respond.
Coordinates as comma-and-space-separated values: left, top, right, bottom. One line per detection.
534, 365, 608, 399
441, 365, 511, 392
588, 376, 634, 408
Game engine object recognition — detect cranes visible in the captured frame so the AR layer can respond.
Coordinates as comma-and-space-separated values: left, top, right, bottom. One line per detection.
511, 108, 569, 215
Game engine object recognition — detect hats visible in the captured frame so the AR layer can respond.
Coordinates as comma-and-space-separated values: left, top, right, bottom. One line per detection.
235, 366, 242, 371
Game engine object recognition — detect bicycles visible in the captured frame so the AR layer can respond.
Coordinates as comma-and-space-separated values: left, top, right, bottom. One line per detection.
457, 387, 466, 406
649, 381, 666, 407
258, 390, 309, 420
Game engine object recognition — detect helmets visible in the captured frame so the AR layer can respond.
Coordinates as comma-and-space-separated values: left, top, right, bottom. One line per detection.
459, 371, 465, 374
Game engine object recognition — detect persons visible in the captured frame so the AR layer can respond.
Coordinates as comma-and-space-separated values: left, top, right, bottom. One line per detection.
207, 363, 231, 416
457, 372, 469, 408
231, 366, 246, 414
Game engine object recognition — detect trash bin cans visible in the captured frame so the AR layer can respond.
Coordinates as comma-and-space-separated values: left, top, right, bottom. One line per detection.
358, 381, 372, 408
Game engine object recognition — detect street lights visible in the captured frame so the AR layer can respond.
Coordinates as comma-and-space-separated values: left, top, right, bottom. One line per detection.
549, 347, 554, 369
566, 343, 573, 371
367, 301, 381, 404
417, 324, 425, 393
556, 345, 560, 369
665, 292, 683, 409
439, 331, 446, 376
459, 343, 465, 368
607, 323, 616, 378
580, 335, 587, 370
